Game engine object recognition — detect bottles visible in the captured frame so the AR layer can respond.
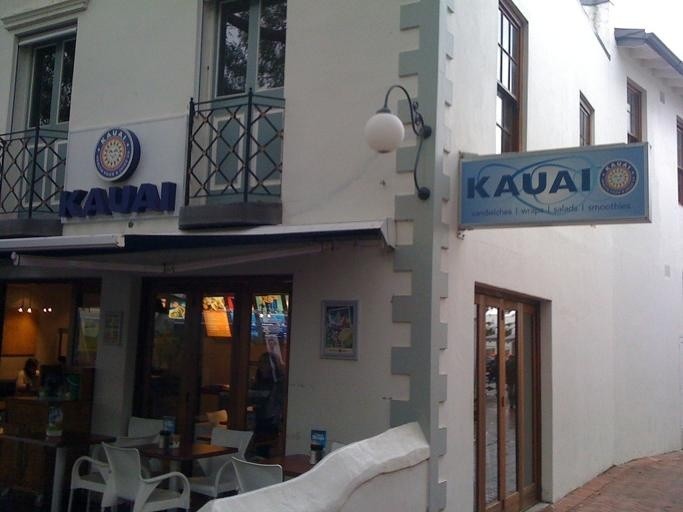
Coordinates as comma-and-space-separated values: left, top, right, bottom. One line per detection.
309, 443, 321, 465
158, 430, 170, 448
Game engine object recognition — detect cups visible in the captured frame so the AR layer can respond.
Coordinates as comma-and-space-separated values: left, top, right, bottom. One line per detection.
169, 433, 180, 448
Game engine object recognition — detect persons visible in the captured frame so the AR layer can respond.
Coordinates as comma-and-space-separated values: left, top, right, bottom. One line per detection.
247, 350, 286, 459
16, 357, 38, 394
149, 336, 208, 434
505, 354, 517, 410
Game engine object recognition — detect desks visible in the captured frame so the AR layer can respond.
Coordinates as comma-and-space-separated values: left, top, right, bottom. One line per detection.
0, 423, 116, 511
256, 454, 313, 477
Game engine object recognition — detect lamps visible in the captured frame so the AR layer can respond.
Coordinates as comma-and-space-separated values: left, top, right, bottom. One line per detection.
365, 83, 432, 202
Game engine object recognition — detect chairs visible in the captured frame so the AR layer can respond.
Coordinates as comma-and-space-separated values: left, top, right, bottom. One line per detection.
67, 405, 284, 511
